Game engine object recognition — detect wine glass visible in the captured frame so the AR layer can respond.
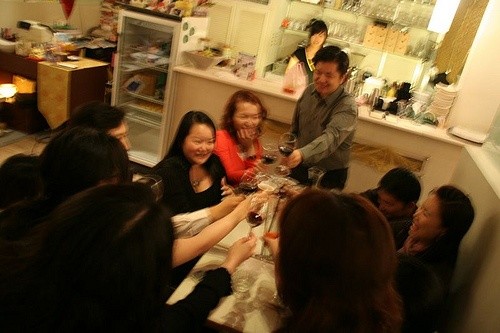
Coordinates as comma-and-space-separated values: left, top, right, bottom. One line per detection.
273, 132, 297, 177
256, 144, 279, 182
245, 191, 269, 240
238, 169, 260, 198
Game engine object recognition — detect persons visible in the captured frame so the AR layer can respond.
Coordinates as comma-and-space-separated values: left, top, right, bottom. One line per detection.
281, 45, 358, 195
150, 109, 235, 222
209, 90, 267, 186
262, 168, 476, 333
284, 21, 328, 92
0, 105, 265, 333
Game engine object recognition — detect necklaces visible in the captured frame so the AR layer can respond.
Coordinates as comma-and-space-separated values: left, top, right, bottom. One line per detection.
190, 175, 204, 189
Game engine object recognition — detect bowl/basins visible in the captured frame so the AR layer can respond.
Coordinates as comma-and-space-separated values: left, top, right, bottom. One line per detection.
53, 51, 71, 61
183, 49, 228, 71
15, 41, 32, 56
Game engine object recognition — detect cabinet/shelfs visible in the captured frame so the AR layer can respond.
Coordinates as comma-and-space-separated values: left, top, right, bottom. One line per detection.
275, 0, 437, 88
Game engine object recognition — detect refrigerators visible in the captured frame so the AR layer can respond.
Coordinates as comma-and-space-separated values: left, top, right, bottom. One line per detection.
111, 9, 210, 168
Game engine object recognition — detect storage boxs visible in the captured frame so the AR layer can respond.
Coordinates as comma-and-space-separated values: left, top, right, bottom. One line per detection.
363, 25, 410, 55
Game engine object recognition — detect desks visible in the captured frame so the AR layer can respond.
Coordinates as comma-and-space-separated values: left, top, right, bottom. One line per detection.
37, 57, 111, 130
166, 201, 292, 333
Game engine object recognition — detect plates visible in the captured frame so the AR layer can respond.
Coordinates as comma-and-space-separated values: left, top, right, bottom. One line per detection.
257, 174, 297, 192
128, 51, 162, 63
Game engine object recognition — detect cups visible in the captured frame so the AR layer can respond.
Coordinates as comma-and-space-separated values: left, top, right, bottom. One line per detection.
263, 186, 287, 240
231, 269, 255, 299
306, 167, 324, 189
135, 177, 159, 202
372, 5, 429, 31
252, 284, 275, 309
345, 66, 379, 109
314, 15, 366, 44
